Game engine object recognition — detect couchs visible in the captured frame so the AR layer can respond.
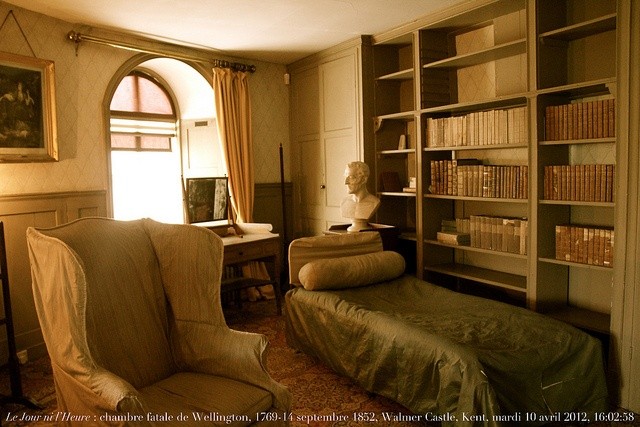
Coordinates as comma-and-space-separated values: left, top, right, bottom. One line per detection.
25, 214, 291, 427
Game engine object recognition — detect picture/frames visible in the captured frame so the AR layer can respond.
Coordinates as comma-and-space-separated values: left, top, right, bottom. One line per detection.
0, 50, 60, 162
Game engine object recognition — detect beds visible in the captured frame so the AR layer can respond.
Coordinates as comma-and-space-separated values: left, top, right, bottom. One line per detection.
282, 229, 608, 425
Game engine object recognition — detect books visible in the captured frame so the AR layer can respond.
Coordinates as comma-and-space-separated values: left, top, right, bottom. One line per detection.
428, 159, 527, 198
555, 225, 614, 268
402, 188, 416, 193
545, 99, 614, 139
542, 163, 614, 203
436, 214, 526, 254
426, 105, 527, 147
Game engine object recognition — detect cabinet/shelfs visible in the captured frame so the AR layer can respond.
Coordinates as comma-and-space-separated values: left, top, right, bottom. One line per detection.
289, 35, 373, 235
418, 0, 532, 308
533, 0, 630, 409
373, 18, 418, 276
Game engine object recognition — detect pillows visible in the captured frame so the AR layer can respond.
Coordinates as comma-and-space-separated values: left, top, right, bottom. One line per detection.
299, 250, 406, 291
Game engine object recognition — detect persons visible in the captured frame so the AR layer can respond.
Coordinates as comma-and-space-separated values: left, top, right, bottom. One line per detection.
339, 161, 380, 220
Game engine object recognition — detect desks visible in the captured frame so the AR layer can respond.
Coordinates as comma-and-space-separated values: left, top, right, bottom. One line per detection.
203, 222, 283, 317
